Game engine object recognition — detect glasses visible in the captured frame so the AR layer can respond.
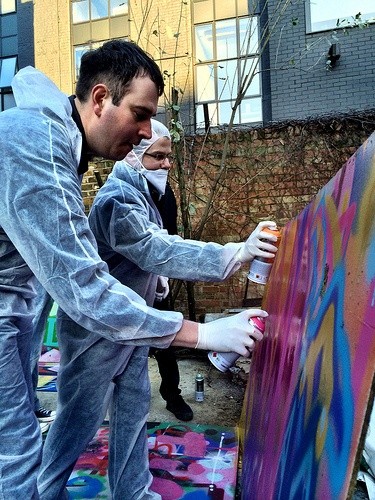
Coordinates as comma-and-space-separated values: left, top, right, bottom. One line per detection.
144, 151, 170, 161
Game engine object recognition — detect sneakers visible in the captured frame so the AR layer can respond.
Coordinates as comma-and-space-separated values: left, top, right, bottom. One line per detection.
39, 423, 49, 433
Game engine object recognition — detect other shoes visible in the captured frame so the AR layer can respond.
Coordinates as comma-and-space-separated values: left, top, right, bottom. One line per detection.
166, 399, 193, 421
34, 407, 57, 421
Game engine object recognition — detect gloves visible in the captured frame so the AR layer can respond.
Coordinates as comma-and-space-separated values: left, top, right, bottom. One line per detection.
154, 275, 170, 301
239, 220, 278, 263
194, 308, 269, 359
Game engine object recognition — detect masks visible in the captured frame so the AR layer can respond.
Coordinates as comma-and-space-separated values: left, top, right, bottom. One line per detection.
126, 151, 170, 195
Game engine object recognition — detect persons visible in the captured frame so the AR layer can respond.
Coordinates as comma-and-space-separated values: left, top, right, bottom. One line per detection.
2, 40, 269, 500
38, 119, 280, 500
141, 183, 195, 421
